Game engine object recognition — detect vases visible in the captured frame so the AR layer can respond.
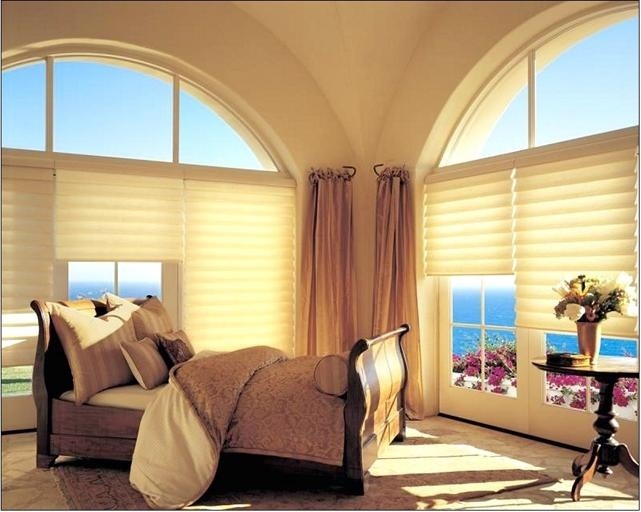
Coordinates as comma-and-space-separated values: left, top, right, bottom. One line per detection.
573, 322, 601, 365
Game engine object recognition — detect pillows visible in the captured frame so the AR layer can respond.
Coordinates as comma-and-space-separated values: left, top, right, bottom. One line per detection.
119, 337, 170, 390
313, 350, 351, 397
46, 301, 141, 406
107, 296, 174, 342
154, 330, 195, 366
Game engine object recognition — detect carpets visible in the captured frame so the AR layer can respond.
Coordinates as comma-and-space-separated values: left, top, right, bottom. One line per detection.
49, 426, 558, 510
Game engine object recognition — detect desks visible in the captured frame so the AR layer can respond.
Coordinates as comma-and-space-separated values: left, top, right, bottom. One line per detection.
531, 355, 641, 502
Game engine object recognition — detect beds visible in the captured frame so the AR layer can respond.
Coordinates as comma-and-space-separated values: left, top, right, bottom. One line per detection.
30, 294, 412, 496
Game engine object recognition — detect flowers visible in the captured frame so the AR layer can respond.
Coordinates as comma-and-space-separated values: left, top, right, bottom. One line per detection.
545, 351, 639, 409
453, 341, 517, 398
552, 274, 630, 322
